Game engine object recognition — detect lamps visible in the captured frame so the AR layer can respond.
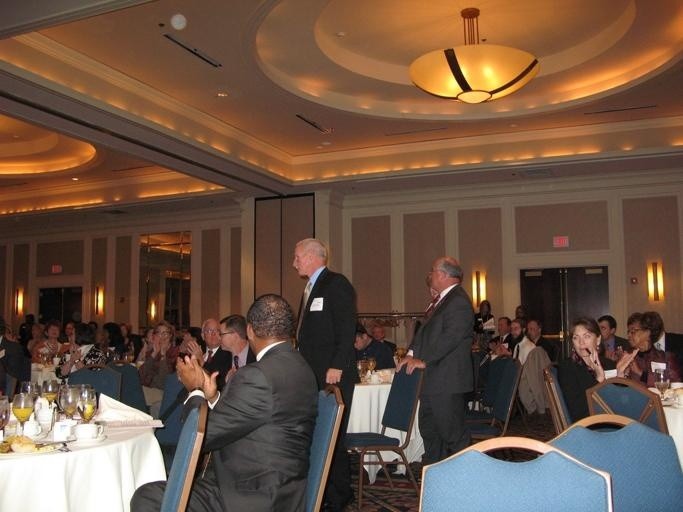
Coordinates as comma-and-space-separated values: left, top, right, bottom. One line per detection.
15, 288, 26, 314
94, 283, 108, 316
645, 259, 667, 304
470, 271, 490, 307
407, 9, 540, 104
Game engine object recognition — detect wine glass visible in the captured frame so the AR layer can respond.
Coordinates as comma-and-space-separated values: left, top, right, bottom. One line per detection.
40, 351, 135, 368
654, 369, 671, 401
355, 348, 407, 385
0, 378, 98, 438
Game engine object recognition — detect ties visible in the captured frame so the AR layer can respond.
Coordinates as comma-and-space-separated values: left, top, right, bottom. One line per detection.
296, 281, 311, 342
234, 356, 238, 369
424, 295, 440, 320
207, 351, 213, 364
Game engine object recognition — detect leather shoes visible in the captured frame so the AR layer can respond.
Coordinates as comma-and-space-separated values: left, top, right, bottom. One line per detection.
320, 493, 355, 512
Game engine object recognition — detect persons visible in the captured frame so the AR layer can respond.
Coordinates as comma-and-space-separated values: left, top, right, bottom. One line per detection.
425, 273, 439, 312
371, 326, 397, 355
289, 239, 355, 511
352, 321, 397, 384
472, 298, 677, 432
397, 257, 477, 465
130, 295, 317, 512
0, 312, 256, 445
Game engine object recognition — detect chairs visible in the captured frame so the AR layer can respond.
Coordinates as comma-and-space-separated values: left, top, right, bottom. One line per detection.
419, 437, 614, 511
160, 395, 209, 511
585, 377, 666, 431
159, 372, 194, 418
307, 385, 345, 511
446, 346, 573, 460
348, 364, 432, 510
116, 364, 154, 414
70, 364, 126, 401
545, 415, 683, 511
